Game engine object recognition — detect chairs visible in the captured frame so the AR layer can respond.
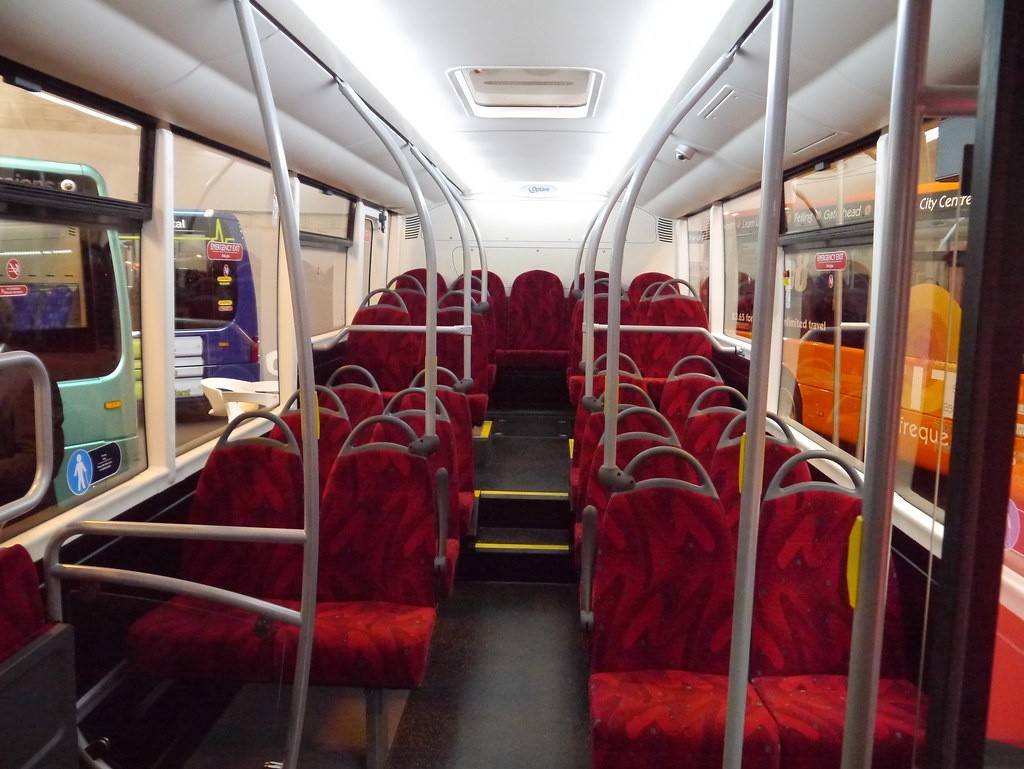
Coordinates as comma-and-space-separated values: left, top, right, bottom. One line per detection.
128, 268, 931, 769
6, 283, 83, 329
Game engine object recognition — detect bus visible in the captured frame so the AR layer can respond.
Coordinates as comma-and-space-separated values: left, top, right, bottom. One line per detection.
0, 154, 142, 536
122, 208, 262, 424
686, 115, 1024, 516
